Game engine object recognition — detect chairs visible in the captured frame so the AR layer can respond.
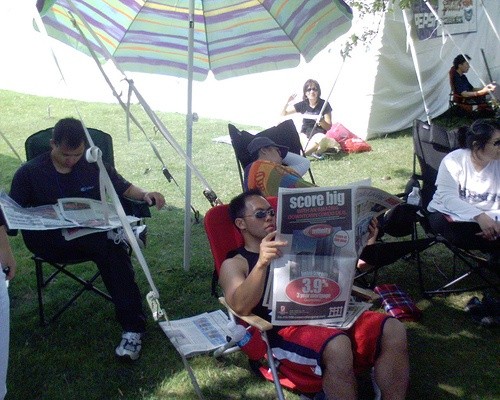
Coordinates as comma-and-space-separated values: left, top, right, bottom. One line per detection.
448, 89, 491, 127
227, 119, 437, 286
408, 120, 500, 298
203, 196, 385, 400
0, 125, 157, 338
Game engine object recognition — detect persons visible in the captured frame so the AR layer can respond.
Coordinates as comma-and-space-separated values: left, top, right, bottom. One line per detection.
427, 118, 500, 254
244, 137, 320, 197
449, 53, 496, 111
219, 187, 409, 399
279, 79, 332, 161
9, 116, 165, 361
0, 206, 16, 400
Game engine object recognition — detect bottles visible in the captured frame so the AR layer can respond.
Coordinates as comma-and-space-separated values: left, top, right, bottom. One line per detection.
407, 187, 421, 206
229, 321, 251, 348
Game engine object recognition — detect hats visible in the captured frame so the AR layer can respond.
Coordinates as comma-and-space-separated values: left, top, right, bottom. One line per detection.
245, 136, 289, 160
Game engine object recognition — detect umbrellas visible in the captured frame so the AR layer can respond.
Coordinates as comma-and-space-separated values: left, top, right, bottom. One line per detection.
32, 0, 353, 271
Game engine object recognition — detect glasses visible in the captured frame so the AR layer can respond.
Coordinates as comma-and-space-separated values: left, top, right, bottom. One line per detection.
305, 88, 317, 91
488, 139, 500, 146
241, 209, 275, 219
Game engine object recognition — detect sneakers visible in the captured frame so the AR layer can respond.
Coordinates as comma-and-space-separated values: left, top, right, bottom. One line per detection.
115, 332, 143, 360
109, 224, 147, 249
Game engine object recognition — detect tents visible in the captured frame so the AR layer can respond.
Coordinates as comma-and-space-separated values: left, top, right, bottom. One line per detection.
0, 0, 500, 141
